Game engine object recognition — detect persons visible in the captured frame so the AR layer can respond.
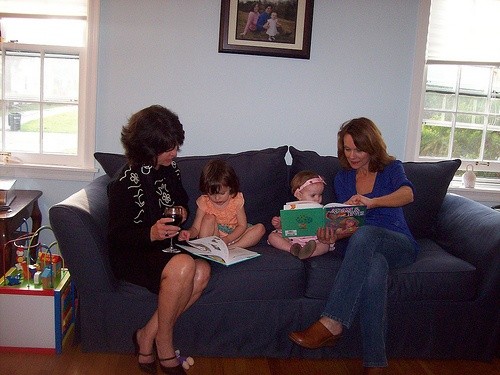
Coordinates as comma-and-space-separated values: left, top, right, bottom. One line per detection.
105, 106, 210, 375
240, 4, 292, 40
179, 159, 266, 246
288, 118, 418, 375
268, 172, 330, 259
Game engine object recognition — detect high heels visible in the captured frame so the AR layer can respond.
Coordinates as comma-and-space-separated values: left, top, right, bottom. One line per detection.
155, 350, 184, 375
133, 329, 157, 375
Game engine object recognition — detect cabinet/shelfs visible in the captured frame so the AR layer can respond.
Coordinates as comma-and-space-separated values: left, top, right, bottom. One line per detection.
0, 267, 73, 353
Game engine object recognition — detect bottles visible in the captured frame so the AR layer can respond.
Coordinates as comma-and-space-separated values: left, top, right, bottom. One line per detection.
461, 163, 476, 189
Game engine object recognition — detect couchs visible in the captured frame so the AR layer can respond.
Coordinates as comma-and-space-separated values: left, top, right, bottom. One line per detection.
48, 146, 500, 363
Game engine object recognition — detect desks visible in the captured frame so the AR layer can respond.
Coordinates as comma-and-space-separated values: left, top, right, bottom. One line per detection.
0, 189, 43, 278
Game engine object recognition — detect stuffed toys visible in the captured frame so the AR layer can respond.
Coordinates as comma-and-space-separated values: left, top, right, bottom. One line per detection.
175, 349, 194, 369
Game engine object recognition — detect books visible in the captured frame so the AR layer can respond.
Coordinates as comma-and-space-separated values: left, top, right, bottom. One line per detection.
176, 236, 262, 266
280, 201, 367, 238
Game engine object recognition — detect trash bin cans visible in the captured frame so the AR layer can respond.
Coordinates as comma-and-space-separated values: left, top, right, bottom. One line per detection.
7, 112, 22, 132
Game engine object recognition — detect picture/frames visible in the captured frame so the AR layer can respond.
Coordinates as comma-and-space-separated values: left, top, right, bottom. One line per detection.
218, 0, 314, 60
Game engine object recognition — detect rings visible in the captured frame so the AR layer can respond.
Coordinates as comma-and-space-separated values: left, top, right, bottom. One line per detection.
165, 230, 169, 236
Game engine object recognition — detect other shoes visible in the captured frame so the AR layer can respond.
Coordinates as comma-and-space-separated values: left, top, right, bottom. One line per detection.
289, 320, 342, 348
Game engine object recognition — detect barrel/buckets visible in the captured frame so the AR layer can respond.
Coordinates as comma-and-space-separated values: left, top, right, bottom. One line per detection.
8, 113, 21, 131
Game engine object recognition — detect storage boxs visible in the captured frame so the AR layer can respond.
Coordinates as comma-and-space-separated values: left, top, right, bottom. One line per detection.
0, 178, 16, 206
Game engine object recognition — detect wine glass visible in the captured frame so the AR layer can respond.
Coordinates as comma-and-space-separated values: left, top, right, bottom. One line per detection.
162, 207, 183, 253
